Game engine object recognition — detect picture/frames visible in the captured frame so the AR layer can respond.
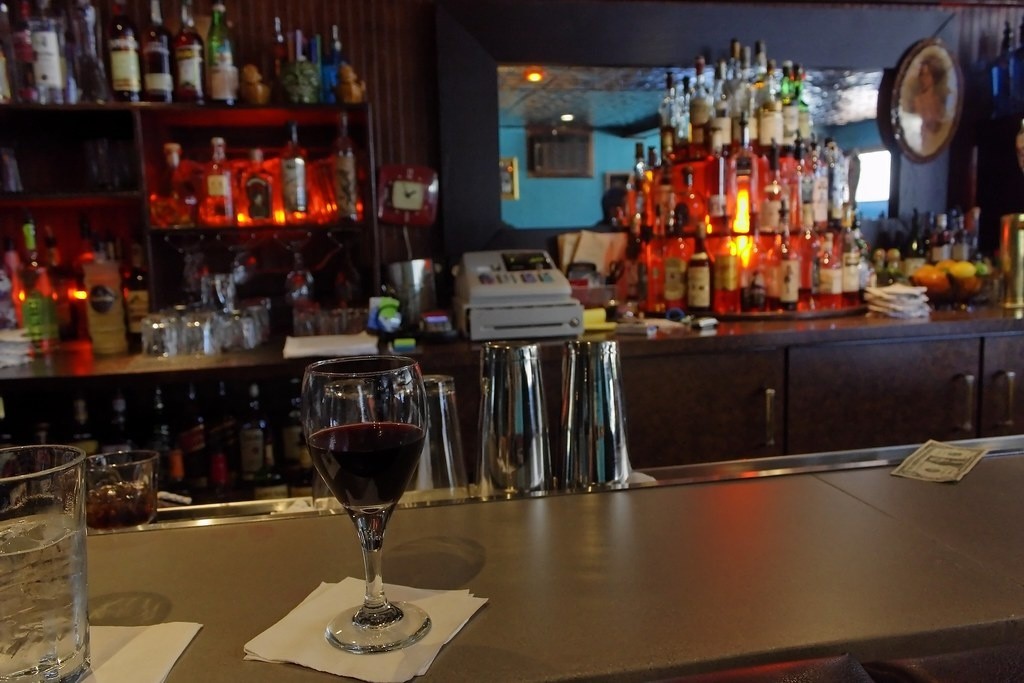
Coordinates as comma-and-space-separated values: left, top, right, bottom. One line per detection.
499, 157, 521, 200
526, 127, 594, 178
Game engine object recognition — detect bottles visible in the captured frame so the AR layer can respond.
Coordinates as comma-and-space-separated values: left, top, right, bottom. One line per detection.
5, 212, 127, 357
149, 114, 358, 224
123, 229, 148, 344
0, 0, 110, 104
141, 0, 174, 102
612, 37, 981, 316
1009, 12, 1024, 113
267, 17, 348, 105
0, 379, 312, 503
172, 0, 203, 103
103, 0, 143, 102
988, 20, 1012, 120
203, 0, 239, 103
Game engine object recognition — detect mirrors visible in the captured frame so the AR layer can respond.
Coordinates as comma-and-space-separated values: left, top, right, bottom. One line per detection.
496, 65, 891, 226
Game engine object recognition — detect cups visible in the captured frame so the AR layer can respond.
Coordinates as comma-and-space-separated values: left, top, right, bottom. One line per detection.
293, 308, 369, 337
407, 375, 470, 498
0, 445, 91, 683
85, 451, 160, 531
139, 305, 271, 359
474, 341, 554, 502
556, 339, 632, 493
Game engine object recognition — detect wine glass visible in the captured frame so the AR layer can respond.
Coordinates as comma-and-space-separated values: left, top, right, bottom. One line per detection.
302, 354, 432, 656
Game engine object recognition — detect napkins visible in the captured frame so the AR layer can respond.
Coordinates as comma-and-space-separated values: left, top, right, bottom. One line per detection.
71, 621, 206, 682
241, 576, 490, 682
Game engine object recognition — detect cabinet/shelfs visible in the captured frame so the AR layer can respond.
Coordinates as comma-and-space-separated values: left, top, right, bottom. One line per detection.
0, 0, 383, 383
426, 302, 1023, 492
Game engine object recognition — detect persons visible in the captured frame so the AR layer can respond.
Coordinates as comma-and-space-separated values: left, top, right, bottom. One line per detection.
591, 188, 642, 262
